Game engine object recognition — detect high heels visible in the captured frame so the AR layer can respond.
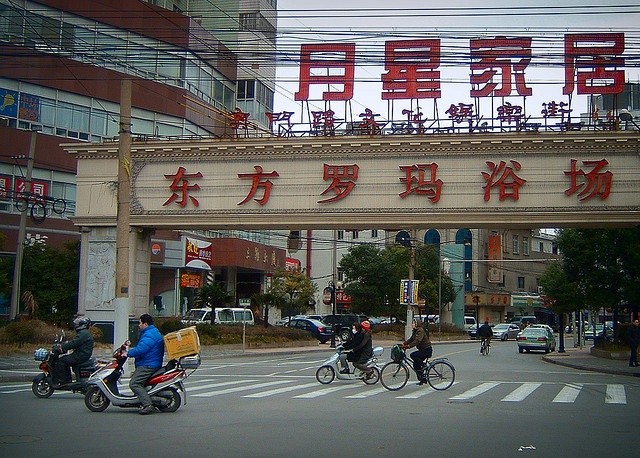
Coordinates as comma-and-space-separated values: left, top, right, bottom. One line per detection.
416, 378, 427, 385
417, 366, 428, 371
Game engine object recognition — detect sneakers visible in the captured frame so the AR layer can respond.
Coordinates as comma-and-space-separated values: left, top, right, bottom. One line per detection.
139, 406, 156, 415
54, 383, 67, 388
366, 371, 373, 379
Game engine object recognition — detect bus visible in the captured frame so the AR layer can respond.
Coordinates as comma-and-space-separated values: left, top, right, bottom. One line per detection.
413, 314, 477, 335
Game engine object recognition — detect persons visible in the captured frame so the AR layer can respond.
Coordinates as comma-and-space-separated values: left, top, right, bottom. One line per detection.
627, 319, 640, 367
121, 314, 164, 414
50, 316, 94, 389
340, 321, 362, 373
479, 321, 493, 355
352, 320, 375, 381
530, 325, 532, 328
525, 323, 530, 329
520, 324, 524, 331
402, 318, 432, 385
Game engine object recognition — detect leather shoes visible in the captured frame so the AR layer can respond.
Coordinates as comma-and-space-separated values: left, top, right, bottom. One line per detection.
340, 368, 349, 374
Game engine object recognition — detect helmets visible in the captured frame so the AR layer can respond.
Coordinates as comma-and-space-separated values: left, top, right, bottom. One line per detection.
73, 317, 90, 330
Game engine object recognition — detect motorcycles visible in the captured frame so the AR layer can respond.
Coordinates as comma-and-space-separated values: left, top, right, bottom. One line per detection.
32, 330, 110, 398
316, 337, 383, 385
84, 340, 200, 412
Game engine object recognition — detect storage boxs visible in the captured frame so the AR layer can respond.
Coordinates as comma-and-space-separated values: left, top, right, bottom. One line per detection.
179, 353, 201, 369
164, 326, 200, 361
373, 347, 383, 356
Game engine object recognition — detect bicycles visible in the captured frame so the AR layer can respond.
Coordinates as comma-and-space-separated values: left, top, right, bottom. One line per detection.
480, 335, 490, 356
380, 344, 456, 391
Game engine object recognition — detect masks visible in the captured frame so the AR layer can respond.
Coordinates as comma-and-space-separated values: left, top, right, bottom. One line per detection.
352, 329, 358, 334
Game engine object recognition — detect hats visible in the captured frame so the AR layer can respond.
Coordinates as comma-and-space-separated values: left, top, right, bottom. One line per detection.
360, 321, 370, 330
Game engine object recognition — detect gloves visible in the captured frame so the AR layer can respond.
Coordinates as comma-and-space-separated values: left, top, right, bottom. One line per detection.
402, 345, 410, 349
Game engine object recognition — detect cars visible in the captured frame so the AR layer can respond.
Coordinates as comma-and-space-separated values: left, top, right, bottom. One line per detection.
565, 321, 589, 333
602, 320, 621, 332
284, 318, 335, 343
275, 316, 293, 327
517, 324, 553, 338
492, 323, 520, 341
369, 317, 406, 332
465, 323, 495, 337
584, 325, 613, 339
516, 328, 556, 353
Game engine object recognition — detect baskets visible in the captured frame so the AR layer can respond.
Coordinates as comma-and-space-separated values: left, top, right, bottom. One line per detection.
34, 351, 49, 361
391, 349, 403, 362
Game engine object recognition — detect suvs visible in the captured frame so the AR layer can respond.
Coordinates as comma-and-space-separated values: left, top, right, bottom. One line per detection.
322, 314, 368, 340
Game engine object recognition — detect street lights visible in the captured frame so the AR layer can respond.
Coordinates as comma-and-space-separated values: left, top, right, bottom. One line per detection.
405, 241, 471, 341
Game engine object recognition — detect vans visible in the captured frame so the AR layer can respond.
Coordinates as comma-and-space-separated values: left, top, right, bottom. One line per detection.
510, 316, 536, 328
181, 307, 254, 325
